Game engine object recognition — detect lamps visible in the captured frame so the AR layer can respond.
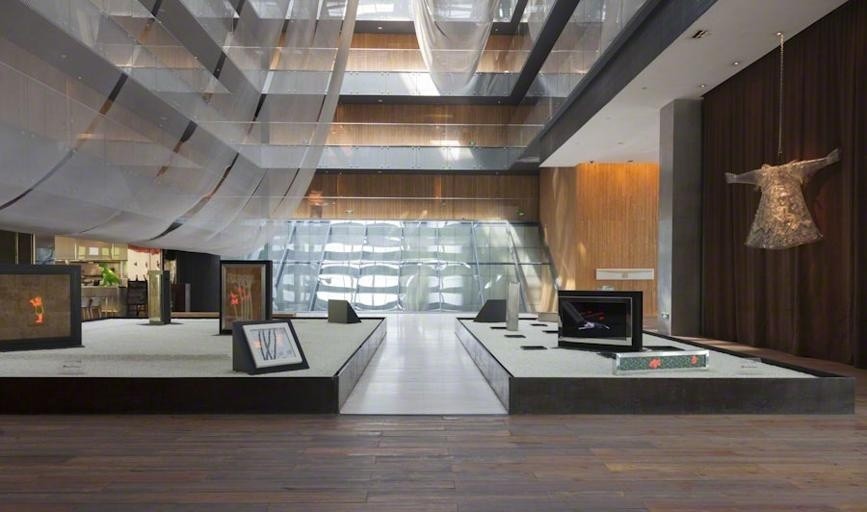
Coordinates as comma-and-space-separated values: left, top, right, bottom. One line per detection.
724, 32, 842, 250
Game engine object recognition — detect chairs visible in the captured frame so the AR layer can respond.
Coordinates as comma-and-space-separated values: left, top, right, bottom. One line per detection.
127, 279, 148, 317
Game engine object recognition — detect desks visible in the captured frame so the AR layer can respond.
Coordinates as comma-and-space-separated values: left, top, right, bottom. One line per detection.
81, 285, 126, 320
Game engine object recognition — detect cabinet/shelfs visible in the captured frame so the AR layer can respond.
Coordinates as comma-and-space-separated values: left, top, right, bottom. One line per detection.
55, 236, 129, 263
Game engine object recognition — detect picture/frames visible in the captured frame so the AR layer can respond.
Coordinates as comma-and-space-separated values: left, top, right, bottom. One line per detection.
0, 263, 82, 352
557, 290, 643, 352
220, 260, 272, 333
